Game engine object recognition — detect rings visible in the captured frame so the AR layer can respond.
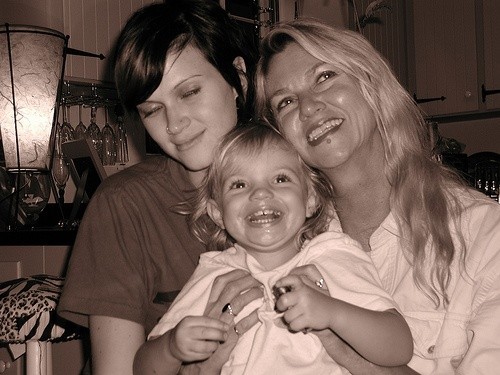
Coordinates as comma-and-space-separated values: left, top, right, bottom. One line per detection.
234, 324, 239, 336
315, 278, 324, 288
221, 302, 236, 317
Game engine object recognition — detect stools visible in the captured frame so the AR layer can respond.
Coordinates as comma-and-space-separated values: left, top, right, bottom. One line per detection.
0, 275, 84, 375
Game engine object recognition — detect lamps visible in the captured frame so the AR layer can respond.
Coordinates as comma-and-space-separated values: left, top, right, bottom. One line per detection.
0, 22, 67, 230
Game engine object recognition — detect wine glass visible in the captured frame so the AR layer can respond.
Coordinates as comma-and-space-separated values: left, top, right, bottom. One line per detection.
52, 155, 69, 202
72, 95, 89, 141
61, 93, 73, 143
99, 98, 116, 165
84, 99, 102, 160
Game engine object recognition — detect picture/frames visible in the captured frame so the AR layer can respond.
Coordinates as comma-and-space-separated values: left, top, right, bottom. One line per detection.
62, 138, 108, 203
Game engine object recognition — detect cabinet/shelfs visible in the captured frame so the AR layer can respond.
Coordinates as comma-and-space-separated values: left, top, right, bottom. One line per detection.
347, 0, 500, 124
0, 245, 85, 375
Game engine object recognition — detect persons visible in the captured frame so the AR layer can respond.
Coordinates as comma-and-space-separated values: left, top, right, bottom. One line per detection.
180, 18, 500, 375
54, 0, 415, 375
